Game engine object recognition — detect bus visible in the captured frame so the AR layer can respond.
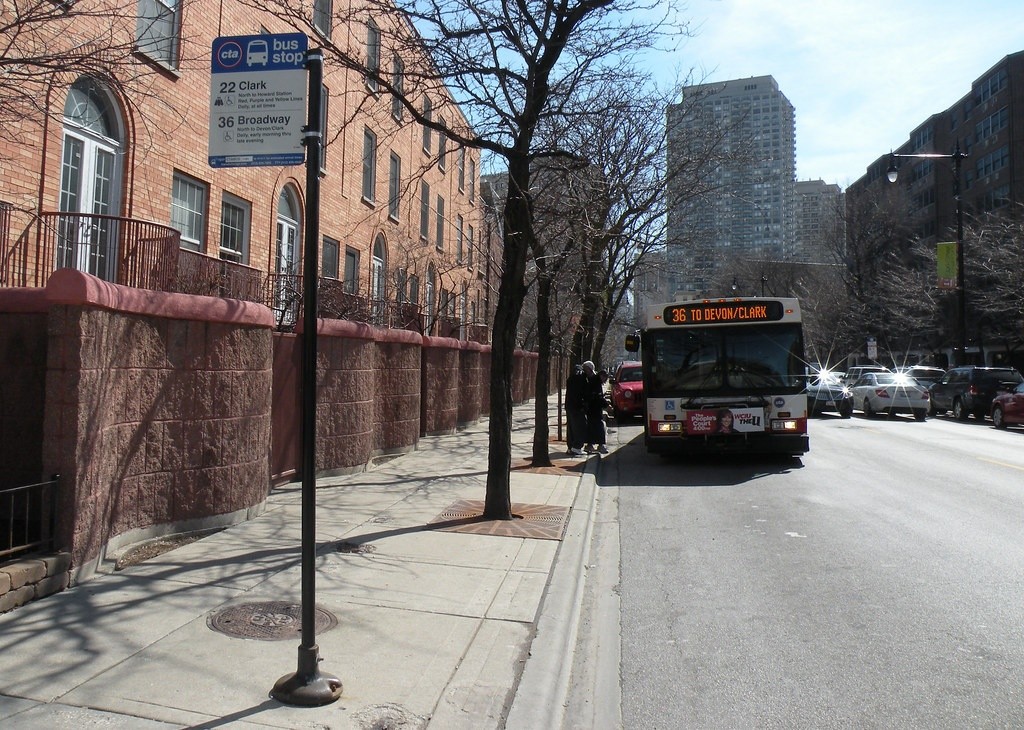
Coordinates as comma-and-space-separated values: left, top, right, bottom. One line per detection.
625, 295, 810, 454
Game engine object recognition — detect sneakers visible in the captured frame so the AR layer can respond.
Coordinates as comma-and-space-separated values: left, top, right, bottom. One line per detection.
584, 445, 597, 454
571, 447, 584, 455
597, 446, 610, 453
566, 449, 578, 455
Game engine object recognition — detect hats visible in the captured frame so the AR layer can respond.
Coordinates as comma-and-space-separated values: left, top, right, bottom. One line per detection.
583, 360, 597, 375
574, 364, 584, 372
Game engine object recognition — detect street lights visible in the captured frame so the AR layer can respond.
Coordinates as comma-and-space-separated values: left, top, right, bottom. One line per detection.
731, 275, 765, 297
887, 149, 967, 365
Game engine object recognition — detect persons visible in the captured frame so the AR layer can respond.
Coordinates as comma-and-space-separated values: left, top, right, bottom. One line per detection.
566, 361, 596, 454
713, 409, 739, 432
584, 369, 613, 453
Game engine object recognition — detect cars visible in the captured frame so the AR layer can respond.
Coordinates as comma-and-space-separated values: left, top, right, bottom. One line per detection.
849, 373, 931, 422
805, 373, 855, 419
989, 381, 1024, 429
609, 360, 646, 422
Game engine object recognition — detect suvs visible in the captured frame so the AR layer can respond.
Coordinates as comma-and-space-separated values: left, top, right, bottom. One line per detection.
839, 365, 893, 390
890, 368, 947, 389
926, 364, 1024, 423
